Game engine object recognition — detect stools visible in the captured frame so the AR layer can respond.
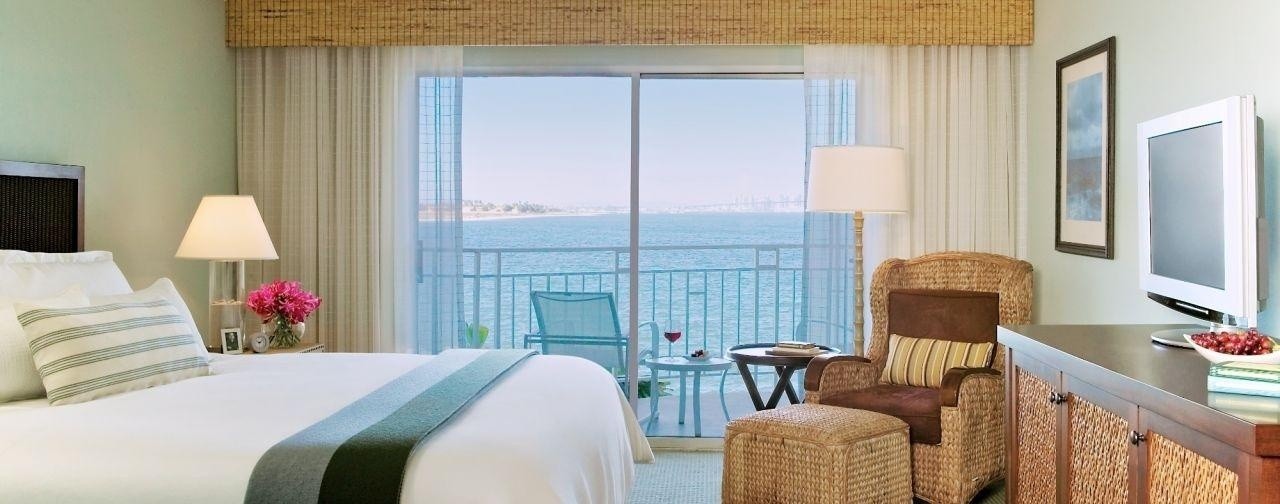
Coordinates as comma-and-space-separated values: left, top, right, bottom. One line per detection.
721, 403, 914, 504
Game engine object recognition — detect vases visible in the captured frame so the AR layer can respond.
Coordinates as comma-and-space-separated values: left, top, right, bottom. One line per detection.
260, 316, 305, 349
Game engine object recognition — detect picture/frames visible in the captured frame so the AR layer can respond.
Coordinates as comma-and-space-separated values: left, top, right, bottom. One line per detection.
220, 328, 243, 355
1056, 36, 1116, 259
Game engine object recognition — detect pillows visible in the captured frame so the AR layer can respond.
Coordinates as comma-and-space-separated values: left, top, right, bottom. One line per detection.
878, 334, 995, 388
0, 284, 91, 401
0, 250, 134, 311
91, 277, 209, 356
16, 297, 220, 407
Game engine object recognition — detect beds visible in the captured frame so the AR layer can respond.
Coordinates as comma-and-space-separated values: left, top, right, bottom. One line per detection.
0, 159, 655, 504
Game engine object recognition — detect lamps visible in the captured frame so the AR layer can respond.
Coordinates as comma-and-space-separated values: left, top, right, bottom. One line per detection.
804, 144, 911, 358
174, 195, 280, 353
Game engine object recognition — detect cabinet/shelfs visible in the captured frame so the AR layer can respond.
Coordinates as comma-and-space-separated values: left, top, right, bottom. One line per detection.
996, 324, 1280, 504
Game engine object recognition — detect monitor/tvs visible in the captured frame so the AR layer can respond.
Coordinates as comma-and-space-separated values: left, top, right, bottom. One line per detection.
1134, 91, 1271, 349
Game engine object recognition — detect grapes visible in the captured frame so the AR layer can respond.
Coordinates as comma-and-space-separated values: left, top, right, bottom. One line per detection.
1191, 328, 1275, 356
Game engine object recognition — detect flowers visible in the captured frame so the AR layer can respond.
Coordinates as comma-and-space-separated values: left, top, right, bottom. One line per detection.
246, 279, 323, 346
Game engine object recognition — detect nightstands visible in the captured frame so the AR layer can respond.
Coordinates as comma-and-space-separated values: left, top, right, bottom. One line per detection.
245, 341, 325, 354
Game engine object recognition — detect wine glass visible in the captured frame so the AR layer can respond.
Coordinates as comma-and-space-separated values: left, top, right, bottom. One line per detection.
663, 318, 682, 363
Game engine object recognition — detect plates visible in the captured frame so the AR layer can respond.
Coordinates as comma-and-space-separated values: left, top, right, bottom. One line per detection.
682, 354, 714, 361
1183, 328, 1280, 366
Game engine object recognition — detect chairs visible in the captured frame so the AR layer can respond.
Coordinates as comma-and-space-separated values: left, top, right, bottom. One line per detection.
524, 290, 659, 426
804, 252, 1034, 504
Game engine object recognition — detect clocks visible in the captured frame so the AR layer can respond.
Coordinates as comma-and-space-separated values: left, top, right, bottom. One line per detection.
249, 332, 270, 353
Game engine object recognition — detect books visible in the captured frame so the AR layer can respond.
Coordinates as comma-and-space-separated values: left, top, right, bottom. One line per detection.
1207, 375, 1280, 398
1210, 361, 1280, 383
1208, 392, 1279, 413
765, 341, 828, 356
